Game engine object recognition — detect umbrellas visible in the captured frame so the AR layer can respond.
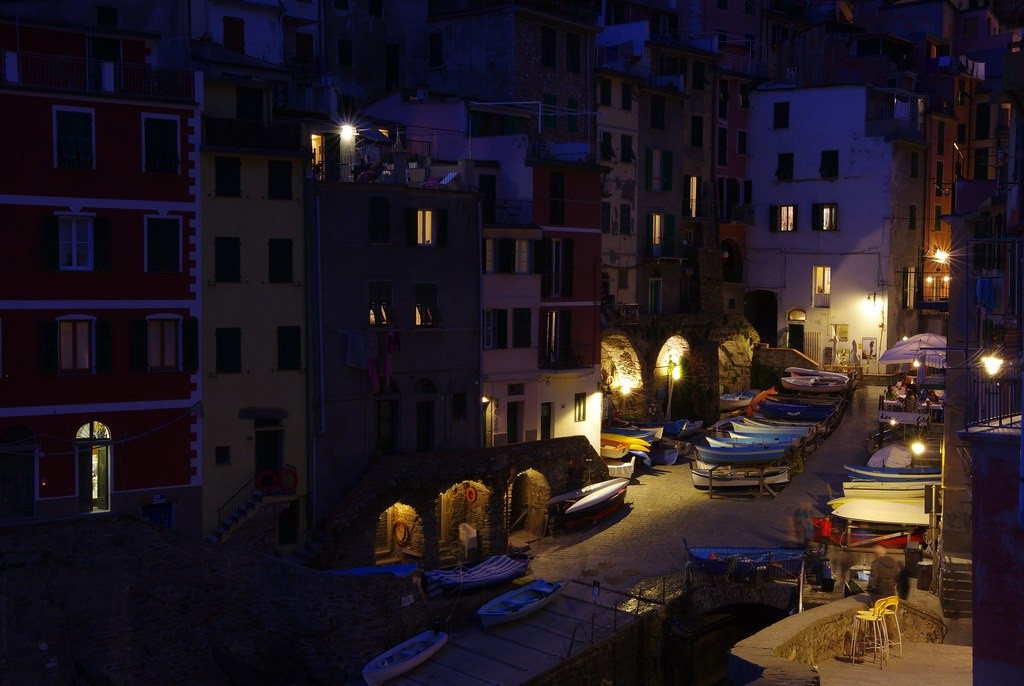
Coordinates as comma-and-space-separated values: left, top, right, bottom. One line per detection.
879, 333, 947, 381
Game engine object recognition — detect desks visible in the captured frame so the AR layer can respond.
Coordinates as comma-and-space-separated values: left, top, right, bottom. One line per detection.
883, 400, 943, 422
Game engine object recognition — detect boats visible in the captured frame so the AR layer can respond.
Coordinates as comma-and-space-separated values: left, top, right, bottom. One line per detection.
547, 476, 630, 531
420, 552, 535, 595
361, 616, 448, 686
600, 365, 942, 582
475, 578, 565, 630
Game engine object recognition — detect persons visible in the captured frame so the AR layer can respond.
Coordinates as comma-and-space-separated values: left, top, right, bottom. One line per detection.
848, 396, 855, 416
867, 546, 900, 649
856, 382, 869, 415
362, 154, 371, 165
898, 384, 920, 413
930, 390, 939, 419
885, 386, 897, 410
895, 376, 907, 390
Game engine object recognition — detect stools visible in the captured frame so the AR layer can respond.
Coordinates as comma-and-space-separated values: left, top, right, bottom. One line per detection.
849, 596, 902, 670
